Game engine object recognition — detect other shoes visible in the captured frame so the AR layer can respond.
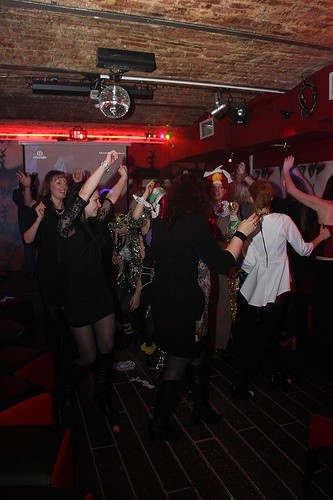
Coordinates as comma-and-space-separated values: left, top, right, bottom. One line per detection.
261, 378, 290, 387
232, 386, 255, 399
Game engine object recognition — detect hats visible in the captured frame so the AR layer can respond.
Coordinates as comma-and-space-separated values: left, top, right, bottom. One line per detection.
149, 183, 166, 204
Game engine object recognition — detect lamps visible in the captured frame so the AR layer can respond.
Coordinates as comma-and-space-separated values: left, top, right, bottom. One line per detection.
209, 89, 250, 125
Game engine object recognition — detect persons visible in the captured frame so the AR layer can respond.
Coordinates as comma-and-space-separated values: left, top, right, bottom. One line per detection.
13, 149, 333, 439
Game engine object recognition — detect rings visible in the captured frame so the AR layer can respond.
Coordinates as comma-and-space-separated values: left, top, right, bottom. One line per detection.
253, 223, 256, 226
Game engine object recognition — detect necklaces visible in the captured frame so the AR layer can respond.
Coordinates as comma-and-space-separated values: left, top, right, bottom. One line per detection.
257, 212, 270, 216
56, 210, 65, 215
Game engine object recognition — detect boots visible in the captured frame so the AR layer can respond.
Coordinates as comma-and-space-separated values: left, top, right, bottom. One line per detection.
93, 353, 114, 388
152, 380, 182, 437
184, 364, 223, 427
73, 363, 89, 388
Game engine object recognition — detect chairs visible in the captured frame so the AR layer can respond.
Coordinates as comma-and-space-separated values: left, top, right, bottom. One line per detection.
0, 299, 94, 500
304, 413, 333, 490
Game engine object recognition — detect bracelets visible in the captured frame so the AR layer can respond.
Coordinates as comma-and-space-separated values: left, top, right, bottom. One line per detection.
132, 195, 152, 207
101, 162, 109, 172
234, 231, 246, 242
